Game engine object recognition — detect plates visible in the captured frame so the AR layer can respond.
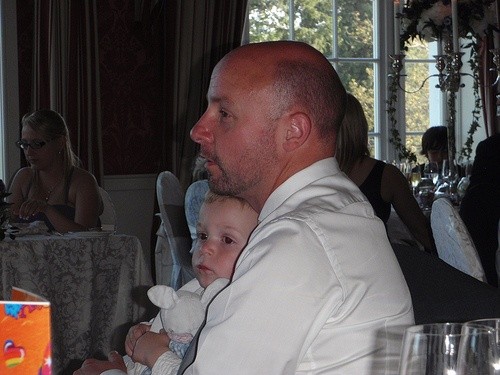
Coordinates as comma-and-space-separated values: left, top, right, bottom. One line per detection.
71, 231, 116, 236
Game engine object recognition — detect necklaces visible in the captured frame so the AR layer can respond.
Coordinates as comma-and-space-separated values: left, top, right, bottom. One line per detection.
38, 175, 63, 200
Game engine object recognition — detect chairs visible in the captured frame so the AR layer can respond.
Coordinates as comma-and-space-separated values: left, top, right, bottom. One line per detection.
155, 170, 211, 291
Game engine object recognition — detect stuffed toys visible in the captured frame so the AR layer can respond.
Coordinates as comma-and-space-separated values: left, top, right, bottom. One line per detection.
147, 278, 230, 361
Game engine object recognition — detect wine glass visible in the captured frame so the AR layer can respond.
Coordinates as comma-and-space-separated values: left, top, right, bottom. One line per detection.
410, 163, 421, 197
442, 160, 458, 195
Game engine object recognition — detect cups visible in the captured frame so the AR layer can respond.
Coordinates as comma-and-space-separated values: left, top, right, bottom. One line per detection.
400, 324, 500, 375
456, 318, 500, 375
424, 160, 438, 185
400, 163, 411, 181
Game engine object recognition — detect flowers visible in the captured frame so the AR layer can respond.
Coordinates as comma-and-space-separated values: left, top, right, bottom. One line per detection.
398, 1, 498, 40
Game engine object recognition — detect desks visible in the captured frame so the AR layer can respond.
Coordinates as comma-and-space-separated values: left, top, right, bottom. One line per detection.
0, 231, 153, 375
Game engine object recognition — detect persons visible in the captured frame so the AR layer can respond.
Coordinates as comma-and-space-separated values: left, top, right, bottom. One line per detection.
74, 40, 415, 375
460, 132, 500, 289
4, 109, 104, 233
121, 192, 260, 375
411, 125, 462, 173
335, 92, 439, 258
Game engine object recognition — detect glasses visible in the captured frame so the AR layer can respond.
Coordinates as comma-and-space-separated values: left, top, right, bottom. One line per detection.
16, 139, 56, 150
